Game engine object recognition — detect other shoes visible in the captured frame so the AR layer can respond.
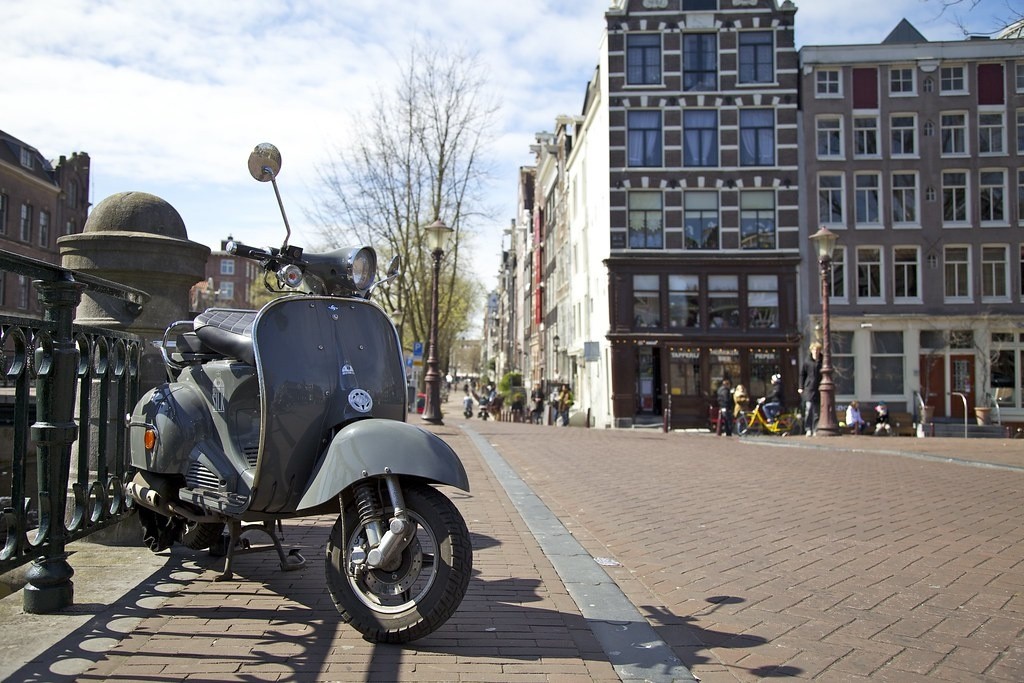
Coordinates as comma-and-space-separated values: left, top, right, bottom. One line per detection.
806, 430, 811, 436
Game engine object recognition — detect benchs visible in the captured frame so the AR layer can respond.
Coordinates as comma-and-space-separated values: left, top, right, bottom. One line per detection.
837, 410, 916, 436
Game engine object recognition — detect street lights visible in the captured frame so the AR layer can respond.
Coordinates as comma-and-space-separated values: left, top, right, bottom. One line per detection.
809, 224, 842, 437
419, 217, 455, 428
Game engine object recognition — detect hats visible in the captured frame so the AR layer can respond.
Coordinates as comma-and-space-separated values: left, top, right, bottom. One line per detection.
771, 374, 782, 383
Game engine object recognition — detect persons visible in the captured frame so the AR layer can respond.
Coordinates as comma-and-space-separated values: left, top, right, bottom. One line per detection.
798, 342, 823, 437
463, 394, 473, 417
873, 401, 894, 436
732, 385, 750, 434
846, 400, 866, 434
479, 395, 489, 417
471, 380, 486, 394
717, 380, 734, 436
447, 382, 468, 395
550, 384, 574, 426
531, 384, 545, 416
489, 394, 505, 421
760, 374, 789, 437
511, 396, 524, 413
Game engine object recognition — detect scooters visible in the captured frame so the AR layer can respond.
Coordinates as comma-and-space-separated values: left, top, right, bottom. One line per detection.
122, 142, 476, 649
464, 408, 474, 420
478, 407, 489, 421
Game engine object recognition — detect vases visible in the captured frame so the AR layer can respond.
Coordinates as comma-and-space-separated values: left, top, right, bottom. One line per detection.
974, 407, 992, 426
918, 405, 934, 423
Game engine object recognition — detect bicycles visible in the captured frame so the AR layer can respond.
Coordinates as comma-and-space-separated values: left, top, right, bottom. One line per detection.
733, 398, 803, 437
